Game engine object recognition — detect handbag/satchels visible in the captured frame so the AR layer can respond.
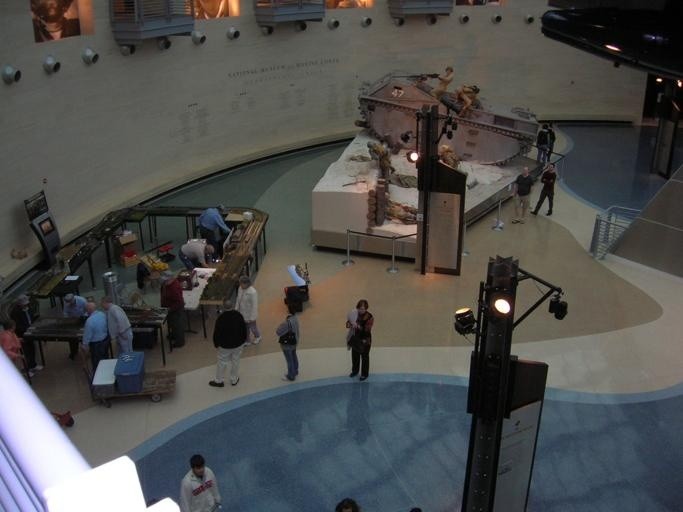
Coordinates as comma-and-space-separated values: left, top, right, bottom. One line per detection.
279, 331, 297, 344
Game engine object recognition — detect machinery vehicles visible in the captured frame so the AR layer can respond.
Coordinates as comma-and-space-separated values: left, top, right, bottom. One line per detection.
355, 69, 540, 168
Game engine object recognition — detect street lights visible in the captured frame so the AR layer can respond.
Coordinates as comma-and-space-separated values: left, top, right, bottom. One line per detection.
444, 254, 570, 512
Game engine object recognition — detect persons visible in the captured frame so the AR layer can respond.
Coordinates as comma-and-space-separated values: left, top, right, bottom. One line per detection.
178, 453, 223, 512
234, 277, 262, 348
80, 301, 110, 372
365, 64, 483, 224
99, 296, 132, 356
208, 297, 246, 386
9, 294, 43, 377
335, 497, 360, 512
344, 300, 373, 380
177, 237, 215, 268
546, 128, 555, 161
510, 166, 535, 223
536, 125, 549, 162
61, 293, 88, 361
0, 318, 29, 372
194, 203, 231, 255
529, 163, 557, 217
159, 270, 189, 347
273, 301, 299, 381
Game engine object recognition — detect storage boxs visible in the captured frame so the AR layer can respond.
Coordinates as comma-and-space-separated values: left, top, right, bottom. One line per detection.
112, 350, 147, 393
91, 358, 120, 396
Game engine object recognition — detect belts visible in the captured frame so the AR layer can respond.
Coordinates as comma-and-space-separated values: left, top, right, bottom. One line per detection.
116, 326, 132, 338
89, 335, 109, 344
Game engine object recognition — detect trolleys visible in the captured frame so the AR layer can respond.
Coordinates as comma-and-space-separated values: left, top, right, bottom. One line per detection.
79, 362, 162, 408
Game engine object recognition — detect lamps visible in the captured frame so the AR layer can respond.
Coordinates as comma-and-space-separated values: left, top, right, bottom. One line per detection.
402, 108, 460, 163
449, 280, 572, 334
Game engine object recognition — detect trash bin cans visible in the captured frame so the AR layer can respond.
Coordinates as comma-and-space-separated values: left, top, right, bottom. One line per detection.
103, 272, 122, 298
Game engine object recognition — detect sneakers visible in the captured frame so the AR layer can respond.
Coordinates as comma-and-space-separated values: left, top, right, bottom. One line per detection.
244, 335, 262, 346
511, 211, 553, 225
349, 372, 369, 381
281, 371, 299, 382
209, 377, 240, 387
